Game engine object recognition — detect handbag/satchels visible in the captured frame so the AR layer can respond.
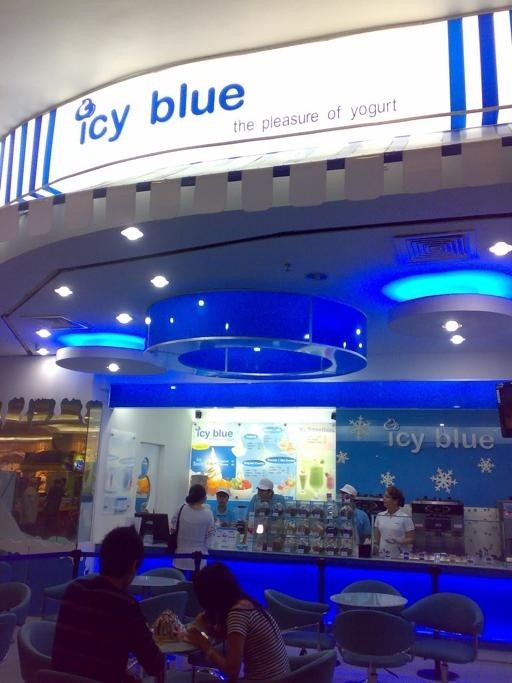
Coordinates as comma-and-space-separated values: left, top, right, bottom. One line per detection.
167, 531, 177, 554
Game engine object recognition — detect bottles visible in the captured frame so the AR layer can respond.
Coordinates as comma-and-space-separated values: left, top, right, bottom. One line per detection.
253, 493, 355, 557
135, 457, 150, 515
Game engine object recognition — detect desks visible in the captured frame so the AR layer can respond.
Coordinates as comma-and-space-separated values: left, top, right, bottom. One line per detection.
331, 591, 408, 612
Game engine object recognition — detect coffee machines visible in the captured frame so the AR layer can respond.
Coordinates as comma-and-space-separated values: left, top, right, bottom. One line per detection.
410, 499, 464, 557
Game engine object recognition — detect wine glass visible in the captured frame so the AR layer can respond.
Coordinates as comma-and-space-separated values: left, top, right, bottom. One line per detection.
298, 471, 308, 494
325, 475, 336, 492
309, 464, 325, 501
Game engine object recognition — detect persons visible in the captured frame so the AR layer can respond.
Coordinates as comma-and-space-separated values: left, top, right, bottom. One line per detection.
184, 560, 292, 682
243, 477, 286, 520
46, 526, 164, 682
211, 485, 238, 526
43, 476, 67, 534
335, 483, 372, 543
374, 486, 416, 557
167, 483, 215, 579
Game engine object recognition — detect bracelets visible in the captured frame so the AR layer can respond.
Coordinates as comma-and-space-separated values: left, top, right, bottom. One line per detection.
204, 645, 216, 660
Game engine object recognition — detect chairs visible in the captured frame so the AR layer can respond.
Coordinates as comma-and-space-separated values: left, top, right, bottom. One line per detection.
2, 612, 18, 661
18, 567, 243, 681
274, 651, 334, 681
264, 588, 330, 652
341, 578, 403, 598
334, 608, 413, 681
1, 581, 32, 626
401, 591, 487, 682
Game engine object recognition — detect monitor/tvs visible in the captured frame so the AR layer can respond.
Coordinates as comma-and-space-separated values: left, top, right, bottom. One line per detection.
134, 512, 169, 544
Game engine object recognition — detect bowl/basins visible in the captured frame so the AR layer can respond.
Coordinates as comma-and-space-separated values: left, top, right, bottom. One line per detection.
231, 482, 253, 499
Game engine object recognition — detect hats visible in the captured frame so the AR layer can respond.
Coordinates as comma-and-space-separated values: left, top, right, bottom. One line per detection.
256, 478, 273, 491
217, 487, 230, 497
340, 484, 357, 499
